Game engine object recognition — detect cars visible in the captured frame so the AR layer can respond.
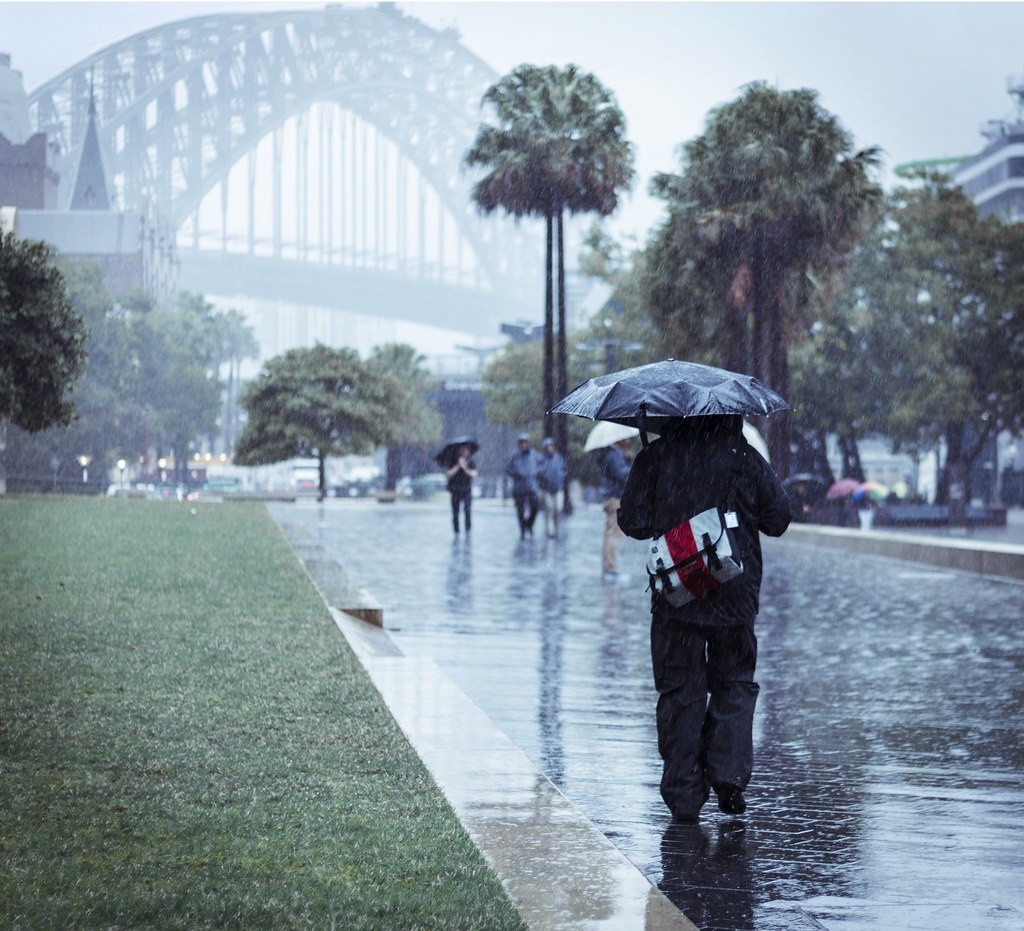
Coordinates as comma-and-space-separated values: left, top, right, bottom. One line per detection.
202, 475, 242, 490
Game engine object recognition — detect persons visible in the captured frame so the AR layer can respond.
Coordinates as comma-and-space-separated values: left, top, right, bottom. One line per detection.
600, 439, 631, 581
982, 463, 995, 510
946, 462, 970, 538
617, 417, 792, 813
507, 435, 567, 538
444, 446, 477, 534
792, 481, 929, 532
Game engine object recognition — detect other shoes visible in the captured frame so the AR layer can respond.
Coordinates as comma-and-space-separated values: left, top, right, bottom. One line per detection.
601, 574, 617, 581
676, 814, 700, 824
714, 783, 747, 814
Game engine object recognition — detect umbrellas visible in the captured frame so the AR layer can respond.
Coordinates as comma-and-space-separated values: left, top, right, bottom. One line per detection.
742, 418, 777, 462
544, 359, 795, 448
434, 435, 481, 465
781, 470, 908, 499
583, 415, 645, 452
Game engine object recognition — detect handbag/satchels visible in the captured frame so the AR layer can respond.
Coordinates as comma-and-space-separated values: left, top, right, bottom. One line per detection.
646, 505, 743, 609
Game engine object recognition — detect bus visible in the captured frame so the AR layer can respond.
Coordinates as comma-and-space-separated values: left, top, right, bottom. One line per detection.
254, 458, 321, 491
